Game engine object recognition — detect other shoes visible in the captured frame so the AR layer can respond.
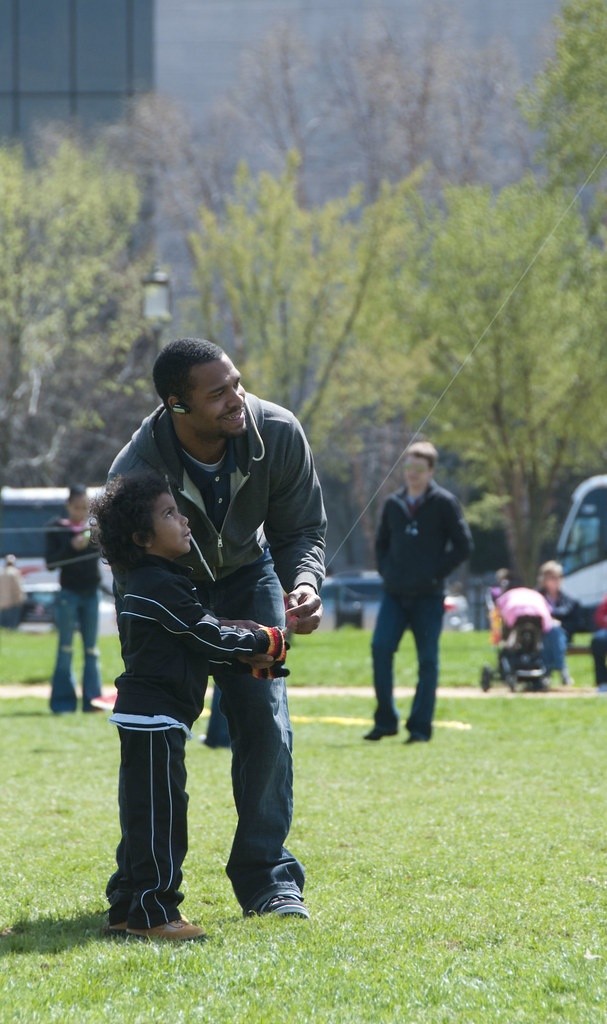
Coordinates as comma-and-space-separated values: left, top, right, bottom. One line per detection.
404, 735, 422, 743
259, 895, 310, 920
364, 728, 398, 740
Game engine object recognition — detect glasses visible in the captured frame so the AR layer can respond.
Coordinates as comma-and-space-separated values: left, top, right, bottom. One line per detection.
403, 462, 429, 472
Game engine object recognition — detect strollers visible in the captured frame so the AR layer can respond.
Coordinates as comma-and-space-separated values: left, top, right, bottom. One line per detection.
480, 587, 549, 691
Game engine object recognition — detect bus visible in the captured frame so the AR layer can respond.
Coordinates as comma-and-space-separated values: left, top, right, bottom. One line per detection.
1, 487, 119, 633
553, 474, 607, 632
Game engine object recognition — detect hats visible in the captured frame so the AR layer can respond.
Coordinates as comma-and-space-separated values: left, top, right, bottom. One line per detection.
91, 686, 118, 711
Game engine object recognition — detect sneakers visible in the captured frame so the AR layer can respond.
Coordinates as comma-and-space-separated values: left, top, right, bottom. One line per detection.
126, 914, 206, 939
109, 921, 128, 930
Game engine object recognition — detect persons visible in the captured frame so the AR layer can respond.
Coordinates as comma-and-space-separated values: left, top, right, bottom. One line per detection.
106, 339, 327, 918
47, 482, 102, 714
442, 583, 469, 629
0, 553, 26, 626
592, 602, 607, 693
486, 560, 579, 687
93, 473, 284, 940
362, 442, 474, 745
